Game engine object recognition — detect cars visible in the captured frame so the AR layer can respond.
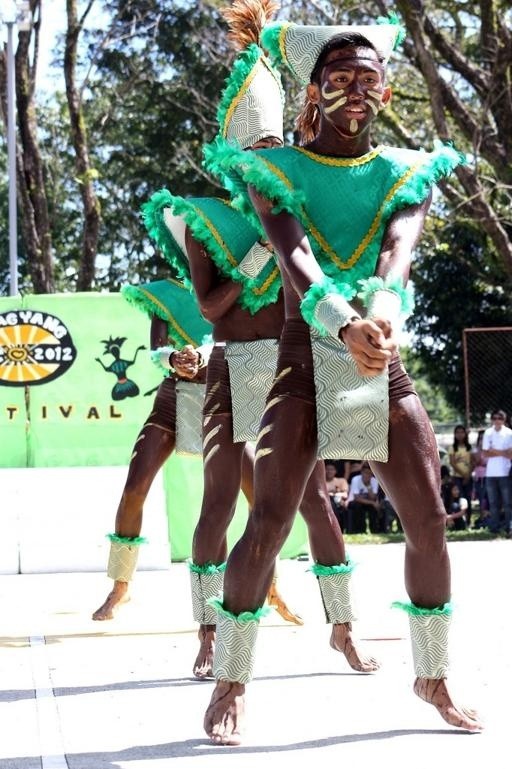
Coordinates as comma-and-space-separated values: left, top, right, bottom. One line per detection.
432, 424, 488, 477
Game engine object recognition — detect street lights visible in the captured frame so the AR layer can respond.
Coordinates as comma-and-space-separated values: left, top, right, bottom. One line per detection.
1, 0, 24, 297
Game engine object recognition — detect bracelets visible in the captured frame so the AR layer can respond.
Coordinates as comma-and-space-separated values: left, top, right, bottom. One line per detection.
230, 239, 274, 283
351, 275, 416, 327
298, 278, 363, 344
196, 341, 215, 369
151, 347, 179, 378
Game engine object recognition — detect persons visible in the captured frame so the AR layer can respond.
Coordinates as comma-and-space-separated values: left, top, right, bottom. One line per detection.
315, 406, 512, 538
201, 16, 485, 749
88, 270, 309, 629
140, 34, 384, 680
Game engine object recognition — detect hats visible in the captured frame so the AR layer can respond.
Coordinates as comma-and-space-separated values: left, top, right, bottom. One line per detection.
263, 22, 405, 85
222, 44, 286, 149
